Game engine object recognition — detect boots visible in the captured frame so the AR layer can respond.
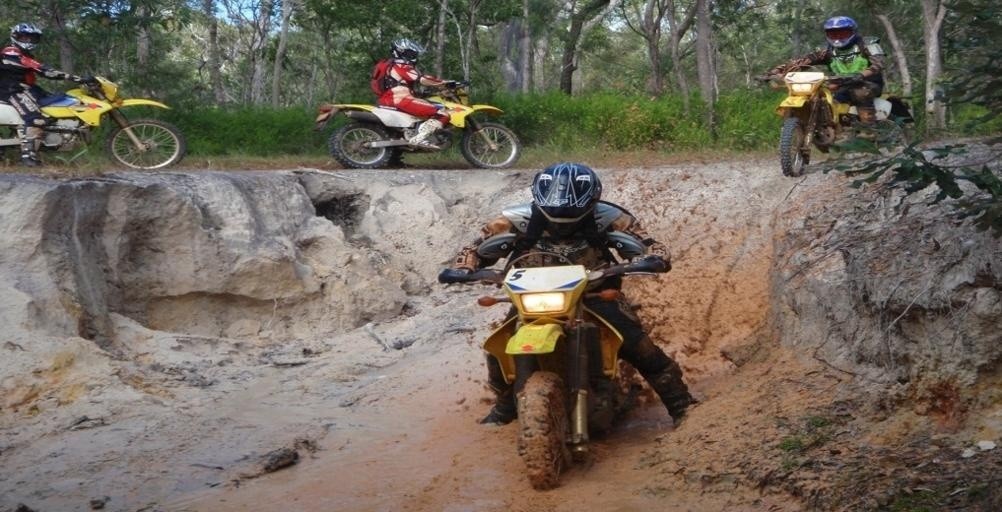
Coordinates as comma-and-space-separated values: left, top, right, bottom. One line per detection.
480, 383, 517, 424
645, 363, 699, 427
410, 119, 443, 150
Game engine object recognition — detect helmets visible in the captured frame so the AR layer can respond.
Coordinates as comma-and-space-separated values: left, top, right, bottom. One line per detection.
10, 23, 42, 51
531, 162, 602, 223
824, 16, 859, 48
391, 38, 425, 65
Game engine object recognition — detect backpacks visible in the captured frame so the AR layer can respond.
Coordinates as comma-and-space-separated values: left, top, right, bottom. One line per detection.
371, 58, 393, 96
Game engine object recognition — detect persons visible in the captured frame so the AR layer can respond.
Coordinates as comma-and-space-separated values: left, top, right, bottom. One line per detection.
368, 36, 461, 150
0, 21, 89, 170
759, 14, 887, 145
438, 160, 702, 430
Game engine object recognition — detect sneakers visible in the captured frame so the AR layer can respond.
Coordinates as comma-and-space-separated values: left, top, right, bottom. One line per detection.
20, 158, 41, 168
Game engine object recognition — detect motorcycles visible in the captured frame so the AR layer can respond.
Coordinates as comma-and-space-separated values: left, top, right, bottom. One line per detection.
751, 65, 914, 178
315, 79, 524, 172
436, 230, 673, 491
1, 72, 186, 174
